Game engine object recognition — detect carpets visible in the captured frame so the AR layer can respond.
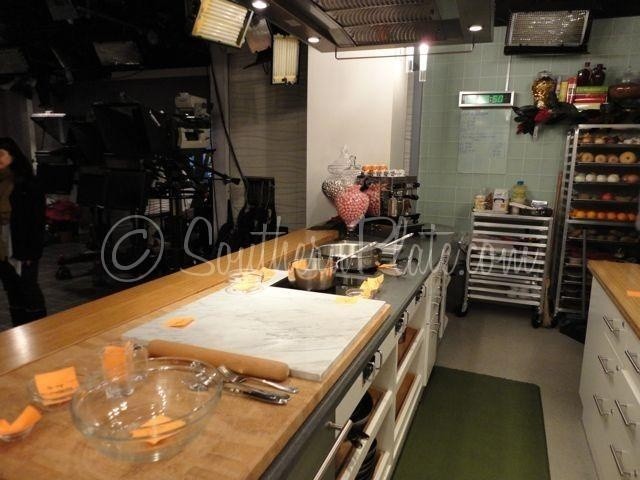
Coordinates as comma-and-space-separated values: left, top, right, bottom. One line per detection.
390, 364, 552, 479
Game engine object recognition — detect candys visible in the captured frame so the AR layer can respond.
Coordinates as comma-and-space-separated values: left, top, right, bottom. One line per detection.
321, 178, 380, 230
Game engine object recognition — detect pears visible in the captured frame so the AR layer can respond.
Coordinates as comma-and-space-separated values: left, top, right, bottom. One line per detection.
581, 150, 636, 164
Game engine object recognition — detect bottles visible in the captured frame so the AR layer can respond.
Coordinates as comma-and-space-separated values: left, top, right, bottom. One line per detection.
474, 195, 486, 212
575, 61, 606, 87
532, 71, 556, 110
510, 180, 528, 205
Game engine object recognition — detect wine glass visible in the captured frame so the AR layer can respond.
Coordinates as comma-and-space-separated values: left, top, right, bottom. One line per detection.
321, 146, 370, 242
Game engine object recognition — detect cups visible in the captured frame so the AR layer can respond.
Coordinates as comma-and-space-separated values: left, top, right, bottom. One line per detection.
0, 397, 43, 443
362, 179, 389, 218
92, 341, 150, 392
568, 257, 582, 265
229, 269, 264, 292
360, 163, 406, 177
26, 369, 91, 414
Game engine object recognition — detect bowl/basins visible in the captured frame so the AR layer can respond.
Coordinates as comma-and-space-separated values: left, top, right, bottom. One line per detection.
353, 438, 378, 480
69, 356, 225, 464
343, 390, 374, 429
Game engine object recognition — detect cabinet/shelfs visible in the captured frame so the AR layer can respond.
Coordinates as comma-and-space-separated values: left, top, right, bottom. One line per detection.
549, 124, 640, 320
277, 248, 449, 480
577, 275, 640, 480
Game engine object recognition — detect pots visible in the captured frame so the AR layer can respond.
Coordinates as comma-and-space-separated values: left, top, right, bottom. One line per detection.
292, 240, 377, 290
316, 232, 414, 270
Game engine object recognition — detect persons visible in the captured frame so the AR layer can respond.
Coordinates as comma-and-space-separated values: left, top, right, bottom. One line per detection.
0, 136, 47, 328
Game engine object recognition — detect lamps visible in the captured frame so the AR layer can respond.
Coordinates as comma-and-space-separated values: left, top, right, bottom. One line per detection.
418, 44, 429, 82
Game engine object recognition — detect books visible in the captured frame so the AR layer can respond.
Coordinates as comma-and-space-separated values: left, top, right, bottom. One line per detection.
554, 80, 608, 112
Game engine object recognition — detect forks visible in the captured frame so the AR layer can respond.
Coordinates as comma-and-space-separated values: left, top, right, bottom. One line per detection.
192, 365, 299, 404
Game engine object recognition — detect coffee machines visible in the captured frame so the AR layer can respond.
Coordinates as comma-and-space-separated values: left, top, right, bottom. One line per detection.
356, 174, 425, 238
